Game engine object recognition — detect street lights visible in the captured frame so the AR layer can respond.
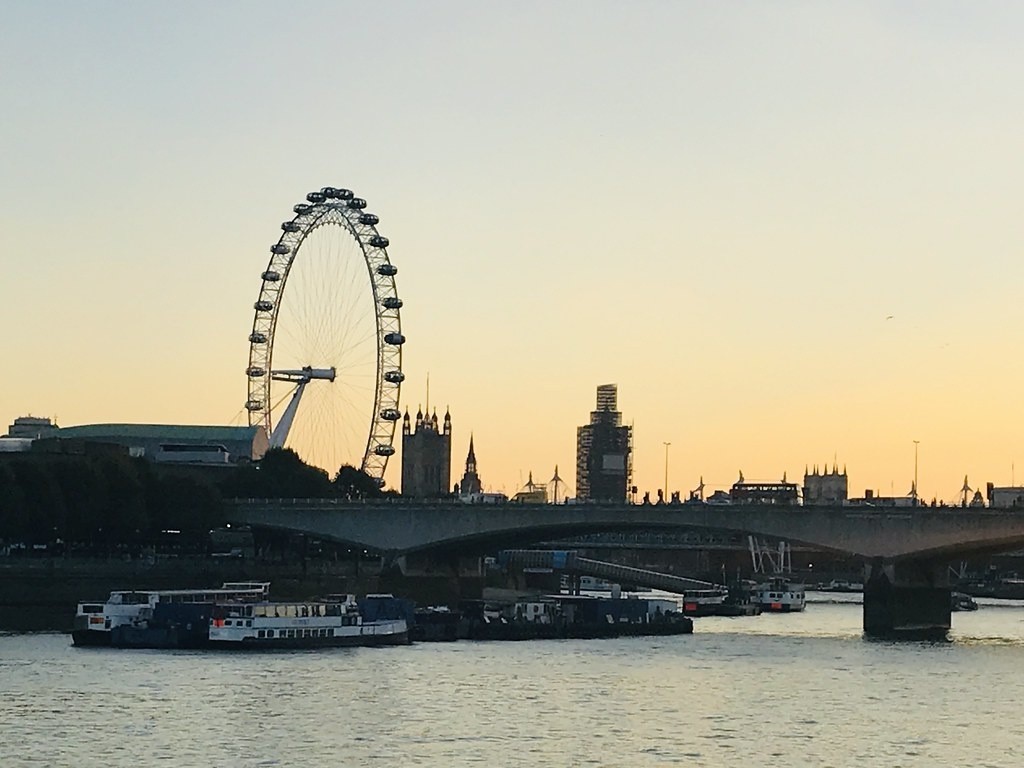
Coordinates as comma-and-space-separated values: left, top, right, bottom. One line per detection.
913, 439, 920, 498
663, 442, 671, 504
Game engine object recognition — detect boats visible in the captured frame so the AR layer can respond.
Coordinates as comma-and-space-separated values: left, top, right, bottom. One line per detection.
71, 588, 264, 649
207, 600, 409, 652
110, 602, 227, 649
762, 572, 807, 612
